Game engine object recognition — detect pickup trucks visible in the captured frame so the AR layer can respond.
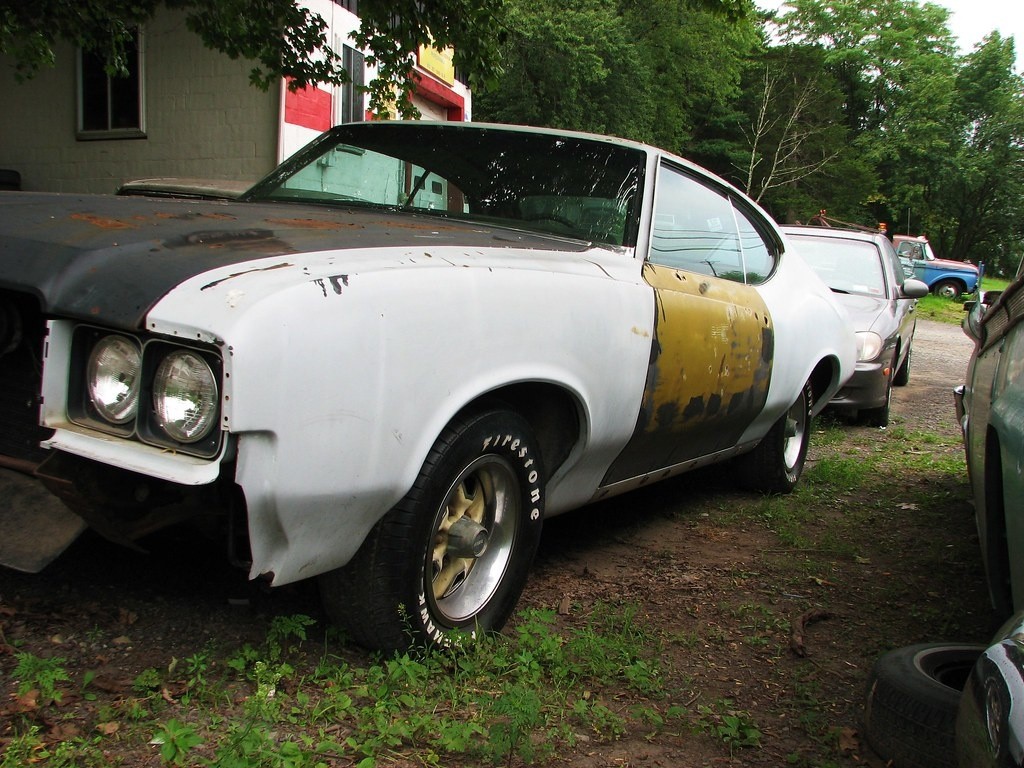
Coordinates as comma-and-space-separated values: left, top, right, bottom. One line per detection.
890, 235, 980, 302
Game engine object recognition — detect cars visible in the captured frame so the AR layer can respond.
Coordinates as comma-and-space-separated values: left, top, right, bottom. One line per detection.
695, 222, 929, 429
854, 251, 1024, 768
0, 116, 864, 668
